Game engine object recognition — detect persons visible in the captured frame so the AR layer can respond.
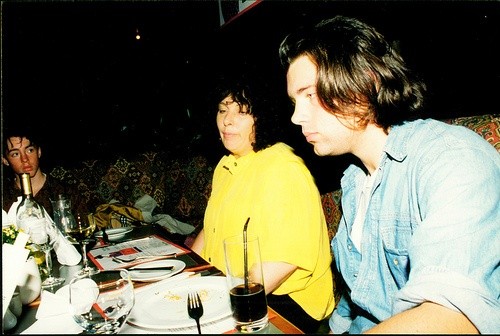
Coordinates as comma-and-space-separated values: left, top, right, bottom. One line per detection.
278, 17, 499, 333
0, 131, 74, 213
191, 79, 334, 332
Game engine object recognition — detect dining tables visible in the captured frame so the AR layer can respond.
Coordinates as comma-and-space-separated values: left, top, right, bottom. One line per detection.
1, 223, 305, 335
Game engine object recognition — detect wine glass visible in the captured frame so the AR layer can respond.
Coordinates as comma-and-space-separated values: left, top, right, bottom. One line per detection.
62, 208, 100, 276
29, 219, 65, 289
69, 275, 133, 336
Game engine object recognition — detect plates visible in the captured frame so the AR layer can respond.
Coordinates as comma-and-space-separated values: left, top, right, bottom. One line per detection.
126, 275, 250, 329
95, 225, 134, 242
120, 259, 186, 283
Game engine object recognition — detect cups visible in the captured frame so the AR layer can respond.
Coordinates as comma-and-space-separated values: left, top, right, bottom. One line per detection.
223, 234, 269, 334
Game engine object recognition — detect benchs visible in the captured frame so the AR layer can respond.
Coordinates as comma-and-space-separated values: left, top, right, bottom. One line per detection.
3, 154, 215, 250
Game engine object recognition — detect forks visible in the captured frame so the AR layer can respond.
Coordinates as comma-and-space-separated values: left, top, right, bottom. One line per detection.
187, 291, 204, 334
